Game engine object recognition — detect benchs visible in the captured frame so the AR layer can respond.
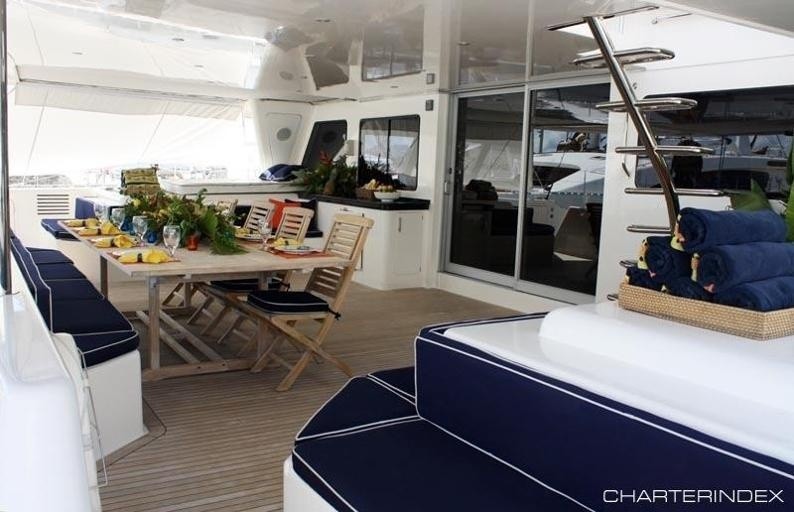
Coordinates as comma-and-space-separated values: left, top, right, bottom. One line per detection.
282, 337, 793, 511
5, 230, 147, 459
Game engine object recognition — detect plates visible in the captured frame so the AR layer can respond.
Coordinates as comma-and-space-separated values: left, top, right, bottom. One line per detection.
234, 232, 275, 243
89, 236, 136, 247
275, 244, 320, 256
111, 247, 170, 258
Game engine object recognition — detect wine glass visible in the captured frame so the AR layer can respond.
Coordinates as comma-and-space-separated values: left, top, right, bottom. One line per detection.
131, 216, 147, 241
162, 226, 180, 259
110, 207, 125, 229
256, 220, 272, 252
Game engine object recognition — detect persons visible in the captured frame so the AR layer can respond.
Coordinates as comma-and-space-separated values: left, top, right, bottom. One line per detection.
659, 134, 707, 188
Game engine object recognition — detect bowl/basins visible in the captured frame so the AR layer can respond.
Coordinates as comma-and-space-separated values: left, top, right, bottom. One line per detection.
373, 191, 401, 203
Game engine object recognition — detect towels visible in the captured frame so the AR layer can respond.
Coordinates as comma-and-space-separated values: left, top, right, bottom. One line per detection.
622, 208, 794, 311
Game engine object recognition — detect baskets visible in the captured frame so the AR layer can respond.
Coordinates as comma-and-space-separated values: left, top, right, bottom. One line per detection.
355, 188, 395, 202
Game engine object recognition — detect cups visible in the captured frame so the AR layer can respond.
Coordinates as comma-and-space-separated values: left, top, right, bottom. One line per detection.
92, 203, 108, 222
